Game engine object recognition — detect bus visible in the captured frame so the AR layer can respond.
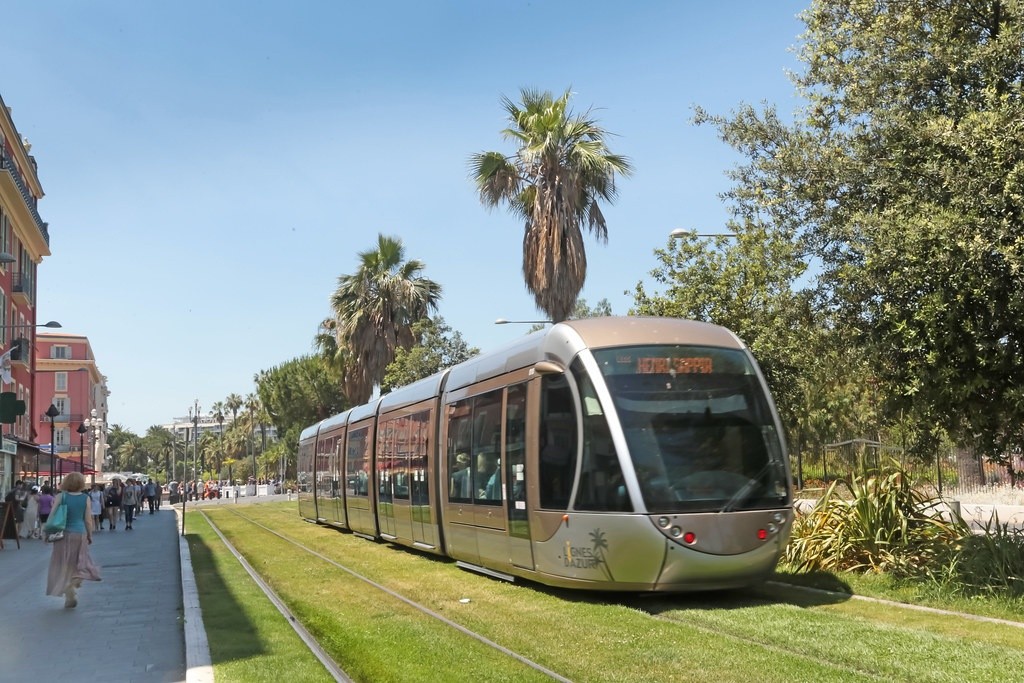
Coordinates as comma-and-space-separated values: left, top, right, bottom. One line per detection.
297, 315, 796, 598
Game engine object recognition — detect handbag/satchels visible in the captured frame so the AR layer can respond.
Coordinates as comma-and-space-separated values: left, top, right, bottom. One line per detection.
44, 492, 67, 533
48, 530, 64, 541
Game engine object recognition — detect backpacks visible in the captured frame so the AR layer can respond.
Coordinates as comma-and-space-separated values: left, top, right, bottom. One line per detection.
5, 488, 20, 502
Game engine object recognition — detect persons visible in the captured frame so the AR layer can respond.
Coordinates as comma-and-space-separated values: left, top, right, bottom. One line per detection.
44, 471, 100, 610
1, 470, 285, 543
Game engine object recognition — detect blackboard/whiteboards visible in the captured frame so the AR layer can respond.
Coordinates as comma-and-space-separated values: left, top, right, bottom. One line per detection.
0, 502, 10, 538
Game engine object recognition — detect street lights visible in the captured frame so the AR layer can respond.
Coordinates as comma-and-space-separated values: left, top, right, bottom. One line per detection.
45, 402, 60, 496
76, 422, 88, 475
83, 409, 104, 487
188, 399, 202, 501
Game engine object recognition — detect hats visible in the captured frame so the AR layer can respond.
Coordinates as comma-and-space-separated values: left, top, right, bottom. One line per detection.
32, 486, 39, 490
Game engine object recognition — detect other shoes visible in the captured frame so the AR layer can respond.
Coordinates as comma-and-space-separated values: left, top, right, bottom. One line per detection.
125, 525, 128, 530
113, 525, 116, 529
100, 527, 104, 530
110, 524, 112, 530
130, 525, 132, 529
65, 600, 77, 608
